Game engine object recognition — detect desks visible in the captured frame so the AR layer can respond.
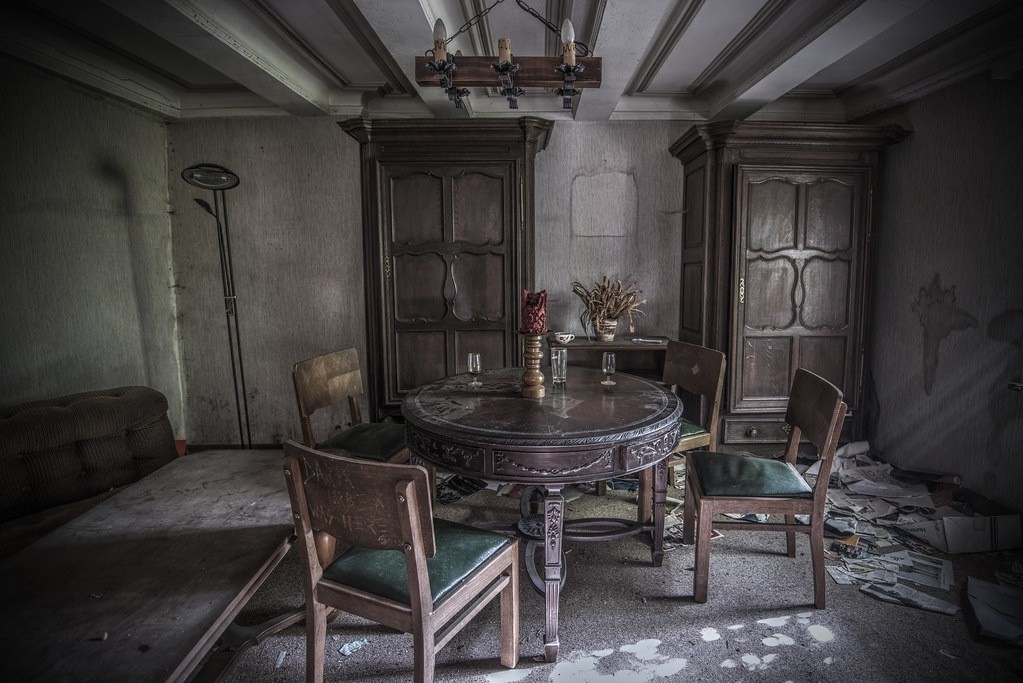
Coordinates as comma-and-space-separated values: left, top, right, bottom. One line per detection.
546, 336, 671, 380
401, 366, 684, 662
0, 449, 339, 683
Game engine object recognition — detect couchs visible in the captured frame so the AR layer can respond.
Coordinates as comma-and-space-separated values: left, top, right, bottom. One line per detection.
0, 386, 181, 561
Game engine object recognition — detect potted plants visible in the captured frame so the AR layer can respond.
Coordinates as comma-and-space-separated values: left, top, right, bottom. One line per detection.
571, 275, 648, 344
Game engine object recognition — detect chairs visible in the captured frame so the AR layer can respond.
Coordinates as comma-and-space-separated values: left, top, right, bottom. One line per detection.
291, 347, 410, 464
595, 340, 727, 534
684, 368, 847, 609
281, 440, 519, 683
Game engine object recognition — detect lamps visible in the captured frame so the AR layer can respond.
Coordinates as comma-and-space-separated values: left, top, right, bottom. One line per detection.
415, 0, 602, 109
181, 162, 255, 449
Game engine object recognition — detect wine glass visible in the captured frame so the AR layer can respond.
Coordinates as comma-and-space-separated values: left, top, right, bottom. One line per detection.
601, 352, 616, 385
468, 353, 483, 385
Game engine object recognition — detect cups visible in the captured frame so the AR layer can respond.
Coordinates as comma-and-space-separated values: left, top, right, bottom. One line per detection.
551, 348, 566, 382
555, 333, 574, 344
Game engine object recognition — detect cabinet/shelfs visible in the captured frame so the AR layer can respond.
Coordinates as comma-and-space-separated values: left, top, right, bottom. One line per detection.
667, 120, 913, 459
